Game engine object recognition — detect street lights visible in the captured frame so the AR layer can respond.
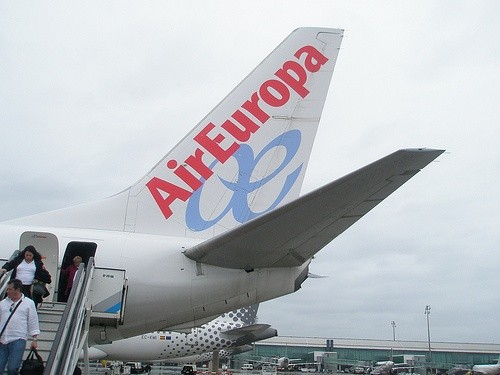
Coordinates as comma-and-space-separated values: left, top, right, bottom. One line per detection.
424, 304, 432, 375
391, 320, 397, 341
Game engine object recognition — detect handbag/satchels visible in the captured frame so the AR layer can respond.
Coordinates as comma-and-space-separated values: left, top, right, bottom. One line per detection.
20, 348, 44, 375
32, 281, 48, 299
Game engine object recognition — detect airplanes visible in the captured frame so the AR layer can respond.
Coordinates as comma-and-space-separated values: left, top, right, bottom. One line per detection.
0, 26, 448, 375
245, 342, 322, 372
357, 347, 418, 374
78, 302, 278, 364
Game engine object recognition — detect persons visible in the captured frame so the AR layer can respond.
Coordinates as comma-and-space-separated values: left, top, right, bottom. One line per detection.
0, 245, 47, 310
61, 256, 82, 298
0, 279, 40, 375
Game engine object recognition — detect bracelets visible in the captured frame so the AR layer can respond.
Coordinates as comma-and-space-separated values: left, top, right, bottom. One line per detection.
33, 338, 38, 341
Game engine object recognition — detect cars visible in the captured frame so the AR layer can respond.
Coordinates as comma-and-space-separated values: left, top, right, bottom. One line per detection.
296, 366, 372, 375
241, 364, 253, 370
181, 365, 197, 375
